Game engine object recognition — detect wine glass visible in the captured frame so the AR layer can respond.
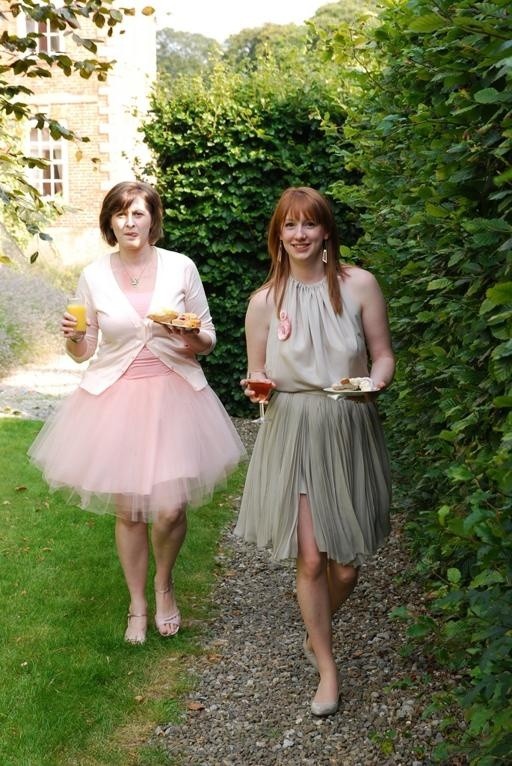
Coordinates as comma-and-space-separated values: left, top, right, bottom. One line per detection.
247, 369, 274, 425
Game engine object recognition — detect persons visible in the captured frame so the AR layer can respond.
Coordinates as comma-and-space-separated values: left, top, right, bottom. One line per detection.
26, 179, 245, 646
228, 184, 396, 718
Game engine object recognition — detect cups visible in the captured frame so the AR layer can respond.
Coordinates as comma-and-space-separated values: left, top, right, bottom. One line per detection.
66, 295, 87, 336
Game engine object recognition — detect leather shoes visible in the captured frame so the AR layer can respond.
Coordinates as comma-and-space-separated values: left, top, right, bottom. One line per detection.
303, 633, 319, 670
309, 675, 340, 716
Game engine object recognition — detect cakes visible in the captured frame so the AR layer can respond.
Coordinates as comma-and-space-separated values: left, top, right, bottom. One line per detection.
331, 375, 375, 392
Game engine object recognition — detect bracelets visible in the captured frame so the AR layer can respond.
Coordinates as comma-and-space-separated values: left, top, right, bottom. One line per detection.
72, 336, 84, 343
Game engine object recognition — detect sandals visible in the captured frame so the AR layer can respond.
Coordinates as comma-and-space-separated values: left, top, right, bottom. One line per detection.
123, 604, 149, 646
154, 578, 181, 637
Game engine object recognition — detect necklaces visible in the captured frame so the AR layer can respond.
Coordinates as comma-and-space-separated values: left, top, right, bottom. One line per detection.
116, 249, 152, 286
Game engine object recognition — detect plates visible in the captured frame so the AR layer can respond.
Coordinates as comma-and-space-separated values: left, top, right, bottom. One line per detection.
153, 320, 200, 334
321, 386, 381, 397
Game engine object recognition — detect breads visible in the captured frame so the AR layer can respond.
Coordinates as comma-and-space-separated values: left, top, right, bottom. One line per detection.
148, 310, 201, 329
